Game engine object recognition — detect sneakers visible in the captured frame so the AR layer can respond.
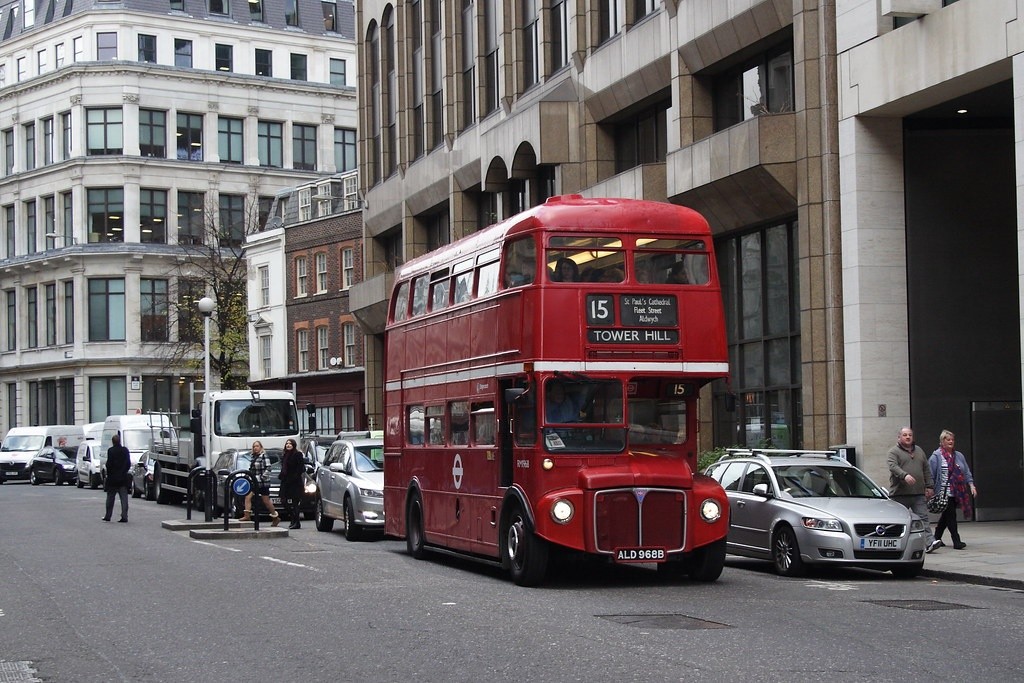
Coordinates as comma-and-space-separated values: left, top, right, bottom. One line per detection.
925, 540, 941, 553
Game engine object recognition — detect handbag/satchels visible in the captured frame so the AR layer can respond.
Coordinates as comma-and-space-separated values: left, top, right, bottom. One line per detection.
927, 491, 948, 514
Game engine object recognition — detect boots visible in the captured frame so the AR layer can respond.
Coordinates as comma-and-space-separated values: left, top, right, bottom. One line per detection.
934, 528, 945, 546
269, 511, 281, 527
290, 519, 300, 529
951, 534, 966, 549
288, 514, 295, 527
239, 510, 253, 521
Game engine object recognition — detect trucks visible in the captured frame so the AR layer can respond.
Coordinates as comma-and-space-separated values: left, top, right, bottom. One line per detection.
146, 390, 317, 511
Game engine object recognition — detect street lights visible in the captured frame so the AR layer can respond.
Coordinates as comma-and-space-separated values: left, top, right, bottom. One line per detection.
199, 298, 214, 521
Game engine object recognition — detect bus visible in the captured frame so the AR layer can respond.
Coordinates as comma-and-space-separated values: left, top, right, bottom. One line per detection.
382, 193, 744, 586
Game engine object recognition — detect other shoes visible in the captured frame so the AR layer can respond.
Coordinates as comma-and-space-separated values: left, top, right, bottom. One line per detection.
118, 519, 128, 523
102, 517, 110, 521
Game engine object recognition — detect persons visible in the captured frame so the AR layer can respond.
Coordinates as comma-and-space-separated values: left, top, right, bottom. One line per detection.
508, 257, 689, 284
887, 427, 941, 553
278, 439, 306, 529
239, 441, 281, 526
101, 435, 131, 522
925, 430, 977, 549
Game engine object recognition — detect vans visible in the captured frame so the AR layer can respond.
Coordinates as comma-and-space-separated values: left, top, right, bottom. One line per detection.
0, 422, 106, 485
100, 414, 178, 492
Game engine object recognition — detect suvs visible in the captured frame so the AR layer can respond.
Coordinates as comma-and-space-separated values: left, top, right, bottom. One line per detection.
703, 448, 926, 579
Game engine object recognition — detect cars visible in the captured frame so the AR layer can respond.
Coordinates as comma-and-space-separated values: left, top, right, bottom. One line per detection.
211, 448, 318, 521
29, 446, 78, 486
300, 430, 385, 541
131, 451, 156, 501
76, 440, 102, 490
